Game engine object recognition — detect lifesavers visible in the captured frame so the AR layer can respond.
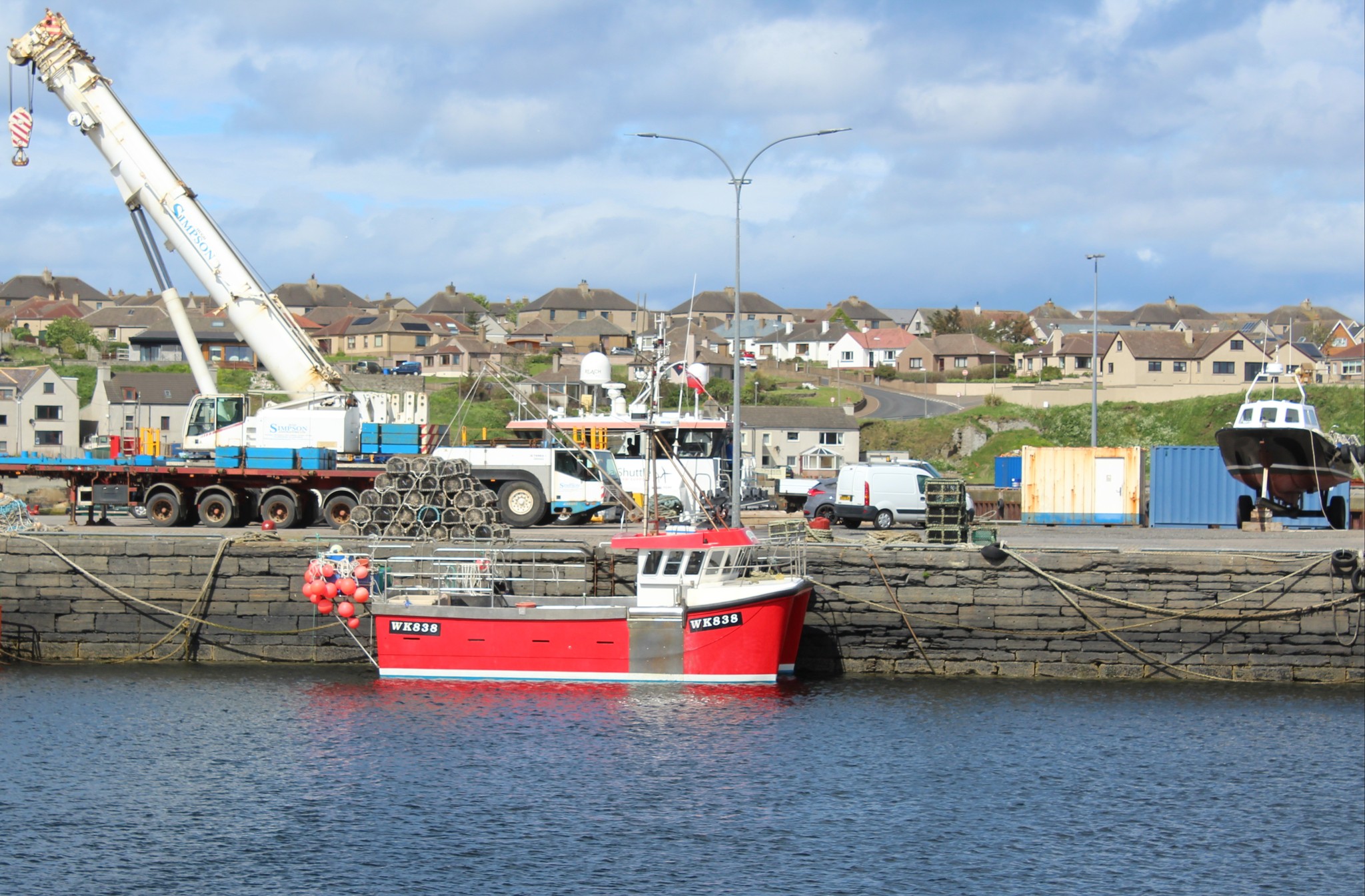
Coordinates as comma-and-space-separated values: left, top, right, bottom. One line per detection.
1356, 445, 1365, 465
1351, 444, 1359, 463
1332, 550, 1357, 566
997, 498, 1004, 509
1351, 568, 1365, 591
1341, 444, 1351, 464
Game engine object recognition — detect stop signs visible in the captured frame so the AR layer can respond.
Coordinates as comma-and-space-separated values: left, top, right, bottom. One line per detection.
955, 391, 961, 398
962, 370, 968, 376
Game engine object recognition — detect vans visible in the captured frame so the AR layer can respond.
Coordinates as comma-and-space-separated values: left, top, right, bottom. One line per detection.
802, 458, 977, 530
390, 361, 422, 375
355, 361, 384, 374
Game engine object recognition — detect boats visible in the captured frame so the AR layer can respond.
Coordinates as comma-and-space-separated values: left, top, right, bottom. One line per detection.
1214, 316, 1357, 499
366, 528, 817, 680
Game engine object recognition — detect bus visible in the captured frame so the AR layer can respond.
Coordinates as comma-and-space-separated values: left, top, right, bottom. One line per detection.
504, 271, 748, 511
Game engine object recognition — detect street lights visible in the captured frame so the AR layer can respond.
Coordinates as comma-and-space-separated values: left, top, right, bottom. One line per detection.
989, 350, 996, 397
1086, 254, 1106, 446
874, 337, 880, 386
754, 381, 758, 406
1038, 350, 1043, 386
920, 367, 927, 416
636, 125, 854, 531
772, 324, 779, 369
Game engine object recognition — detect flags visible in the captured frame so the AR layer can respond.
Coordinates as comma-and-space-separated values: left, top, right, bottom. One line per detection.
685, 370, 705, 394
672, 364, 684, 376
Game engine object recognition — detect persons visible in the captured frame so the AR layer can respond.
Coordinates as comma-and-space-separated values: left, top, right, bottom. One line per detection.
627, 438, 637, 456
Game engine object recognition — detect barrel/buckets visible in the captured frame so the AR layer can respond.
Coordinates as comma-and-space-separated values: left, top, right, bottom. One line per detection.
369, 564, 392, 592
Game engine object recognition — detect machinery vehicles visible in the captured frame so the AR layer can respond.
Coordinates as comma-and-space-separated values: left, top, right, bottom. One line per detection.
0, 8, 628, 531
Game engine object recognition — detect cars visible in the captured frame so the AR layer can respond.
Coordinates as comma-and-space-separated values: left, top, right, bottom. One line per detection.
610, 344, 639, 356
773, 465, 794, 478
738, 349, 757, 368
1, 352, 11, 362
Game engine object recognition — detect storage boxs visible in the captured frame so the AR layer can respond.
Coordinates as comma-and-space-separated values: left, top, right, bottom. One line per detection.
751, 472, 819, 495
215, 422, 449, 470
